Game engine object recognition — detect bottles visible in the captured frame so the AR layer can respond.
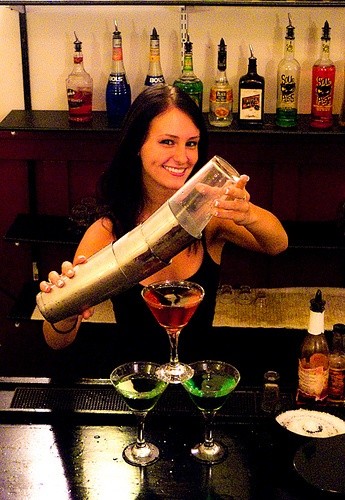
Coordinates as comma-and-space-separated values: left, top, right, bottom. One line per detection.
142, 26, 166, 91
309, 20, 336, 129
295, 289, 328, 409
328, 324, 345, 401
64, 30, 94, 122
261, 370, 282, 413
105, 19, 132, 126
173, 34, 204, 113
276, 13, 300, 127
208, 37, 234, 127
237, 43, 265, 129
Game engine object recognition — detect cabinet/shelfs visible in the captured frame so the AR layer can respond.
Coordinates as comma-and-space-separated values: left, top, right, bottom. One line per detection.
1, 110, 345, 322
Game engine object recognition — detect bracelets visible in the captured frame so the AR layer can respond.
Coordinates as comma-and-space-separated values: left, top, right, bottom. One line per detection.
50, 317, 78, 334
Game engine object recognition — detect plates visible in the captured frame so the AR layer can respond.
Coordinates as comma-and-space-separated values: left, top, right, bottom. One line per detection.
275, 409, 345, 438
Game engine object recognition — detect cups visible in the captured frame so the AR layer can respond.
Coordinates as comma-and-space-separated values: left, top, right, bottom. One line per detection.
142, 199, 202, 262
168, 155, 241, 237
113, 222, 172, 284
36, 240, 135, 324
219, 284, 269, 308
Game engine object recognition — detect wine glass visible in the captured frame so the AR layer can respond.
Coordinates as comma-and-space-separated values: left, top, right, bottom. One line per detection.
179, 360, 241, 465
141, 279, 205, 384
110, 361, 171, 467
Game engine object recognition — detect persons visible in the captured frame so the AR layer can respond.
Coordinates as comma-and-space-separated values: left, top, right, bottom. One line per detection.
39, 84, 289, 351
